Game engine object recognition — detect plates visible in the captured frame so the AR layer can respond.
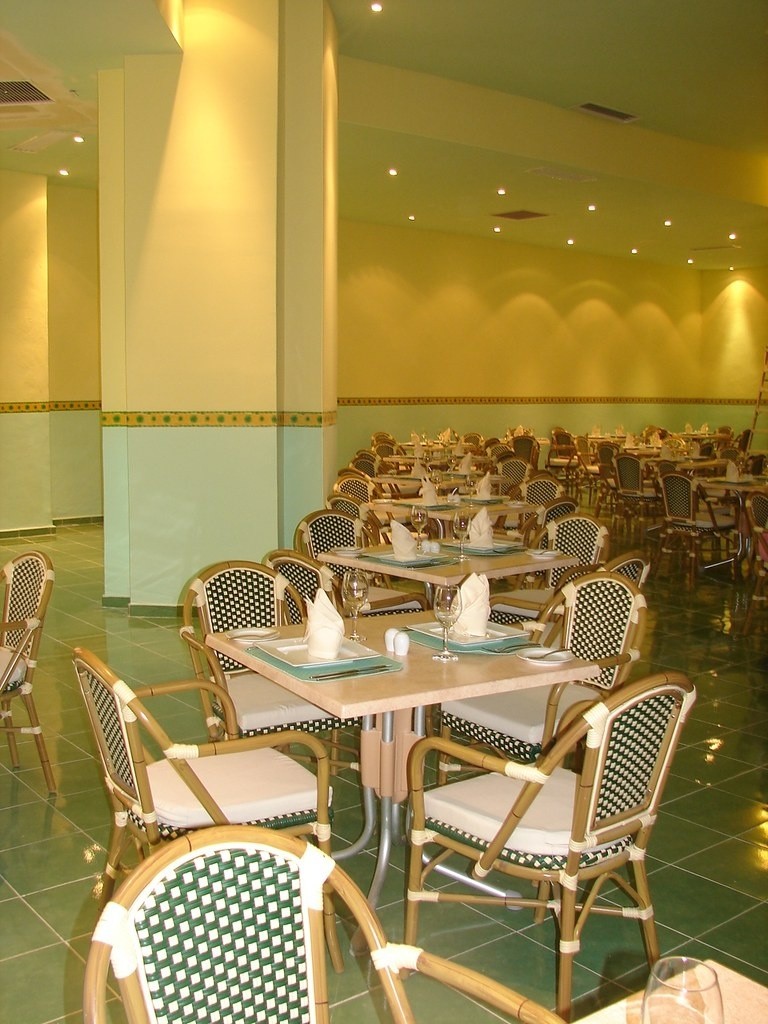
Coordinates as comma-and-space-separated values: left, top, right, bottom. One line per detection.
405, 620, 530, 647
331, 547, 364, 558
526, 551, 563, 560
225, 628, 280, 645
443, 539, 523, 553
397, 499, 456, 510
253, 635, 381, 668
516, 646, 574, 666
461, 496, 510, 505
362, 550, 449, 565
373, 499, 396, 506
504, 501, 529, 507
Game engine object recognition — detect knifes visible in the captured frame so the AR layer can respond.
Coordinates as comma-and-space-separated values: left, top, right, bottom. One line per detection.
315, 669, 389, 681
405, 561, 459, 569
311, 664, 393, 681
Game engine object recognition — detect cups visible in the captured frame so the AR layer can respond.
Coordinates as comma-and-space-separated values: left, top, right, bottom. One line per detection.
644, 957, 724, 1024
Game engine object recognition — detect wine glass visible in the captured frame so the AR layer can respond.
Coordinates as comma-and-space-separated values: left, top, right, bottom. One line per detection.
426, 439, 433, 455
688, 442, 696, 463
422, 433, 426, 443
447, 454, 457, 478
411, 505, 428, 556
431, 470, 443, 496
736, 457, 745, 479
342, 571, 369, 643
465, 474, 477, 508
453, 512, 471, 561
423, 453, 433, 474
434, 585, 462, 663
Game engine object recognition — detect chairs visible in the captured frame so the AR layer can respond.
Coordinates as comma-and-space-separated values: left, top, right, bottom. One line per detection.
0, 425, 768, 1024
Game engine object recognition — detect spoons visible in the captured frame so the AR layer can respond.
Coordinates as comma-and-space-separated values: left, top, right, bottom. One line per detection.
482, 644, 541, 653
228, 631, 279, 640
493, 549, 528, 554
527, 648, 572, 660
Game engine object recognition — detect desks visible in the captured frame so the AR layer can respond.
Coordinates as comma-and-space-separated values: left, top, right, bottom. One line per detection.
384, 455, 490, 474
693, 474, 768, 586
318, 537, 578, 610
204, 606, 600, 959
359, 496, 540, 539
638, 456, 729, 481
584, 433, 637, 453
568, 960, 768, 1024
496, 437, 550, 453
622, 446, 690, 481
677, 432, 730, 445
398, 438, 473, 459
371, 473, 505, 497
